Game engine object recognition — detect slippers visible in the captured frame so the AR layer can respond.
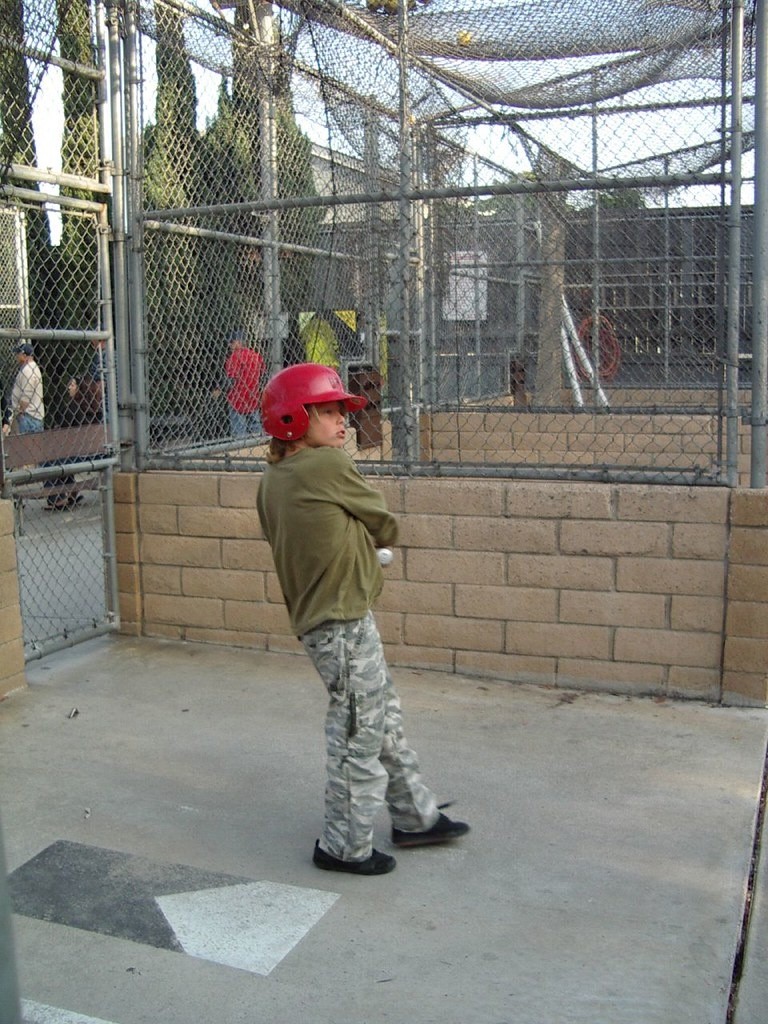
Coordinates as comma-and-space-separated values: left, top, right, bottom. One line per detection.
41, 495, 84, 510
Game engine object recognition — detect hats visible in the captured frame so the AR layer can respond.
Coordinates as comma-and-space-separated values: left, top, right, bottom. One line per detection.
12, 344, 34, 356
231, 331, 246, 340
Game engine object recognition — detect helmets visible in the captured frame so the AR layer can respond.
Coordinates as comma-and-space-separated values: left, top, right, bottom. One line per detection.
262, 363, 367, 441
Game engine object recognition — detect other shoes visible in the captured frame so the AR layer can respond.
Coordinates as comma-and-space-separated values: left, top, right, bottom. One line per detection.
391, 800, 469, 847
312, 839, 396, 875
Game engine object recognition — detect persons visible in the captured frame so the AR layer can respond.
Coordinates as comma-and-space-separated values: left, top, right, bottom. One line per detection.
10, 344, 44, 435
93, 328, 118, 412
256, 364, 470, 875
212, 331, 262, 438
43, 353, 84, 510
